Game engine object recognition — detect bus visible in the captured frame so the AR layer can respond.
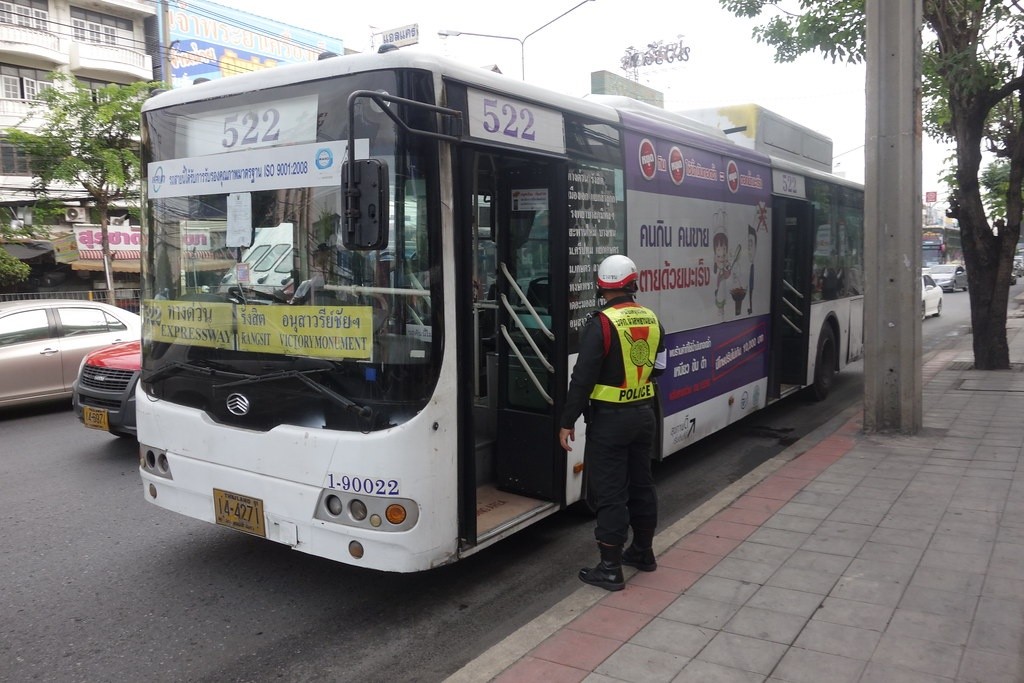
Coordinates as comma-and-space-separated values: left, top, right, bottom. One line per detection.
922, 224, 963, 268
133, 45, 865, 572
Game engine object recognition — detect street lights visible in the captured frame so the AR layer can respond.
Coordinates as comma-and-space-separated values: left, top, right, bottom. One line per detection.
435, 27, 525, 81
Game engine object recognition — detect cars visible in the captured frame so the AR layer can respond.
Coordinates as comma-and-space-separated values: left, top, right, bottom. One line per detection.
0, 298, 142, 406
920, 274, 945, 321
928, 264, 967, 292
1010, 255, 1024, 288
72, 339, 141, 436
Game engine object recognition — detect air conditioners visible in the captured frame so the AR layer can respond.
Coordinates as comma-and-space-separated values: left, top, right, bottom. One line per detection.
65, 207, 86, 222
110, 217, 129, 226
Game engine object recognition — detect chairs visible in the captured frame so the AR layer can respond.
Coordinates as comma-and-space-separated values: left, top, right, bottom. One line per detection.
486, 280, 524, 307
524, 276, 549, 309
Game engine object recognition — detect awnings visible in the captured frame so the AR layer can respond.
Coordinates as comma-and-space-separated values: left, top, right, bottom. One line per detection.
67, 260, 238, 273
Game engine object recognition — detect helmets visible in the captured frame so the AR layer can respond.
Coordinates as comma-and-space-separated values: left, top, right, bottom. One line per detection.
597, 255, 638, 289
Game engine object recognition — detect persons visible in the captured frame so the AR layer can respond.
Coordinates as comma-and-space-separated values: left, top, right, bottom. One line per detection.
818, 249, 844, 300
285, 248, 359, 306
559, 254, 665, 590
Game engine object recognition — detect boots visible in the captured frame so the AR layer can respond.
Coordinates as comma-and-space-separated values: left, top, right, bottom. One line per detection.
579, 542, 625, 590
622, 529, 656, 570
